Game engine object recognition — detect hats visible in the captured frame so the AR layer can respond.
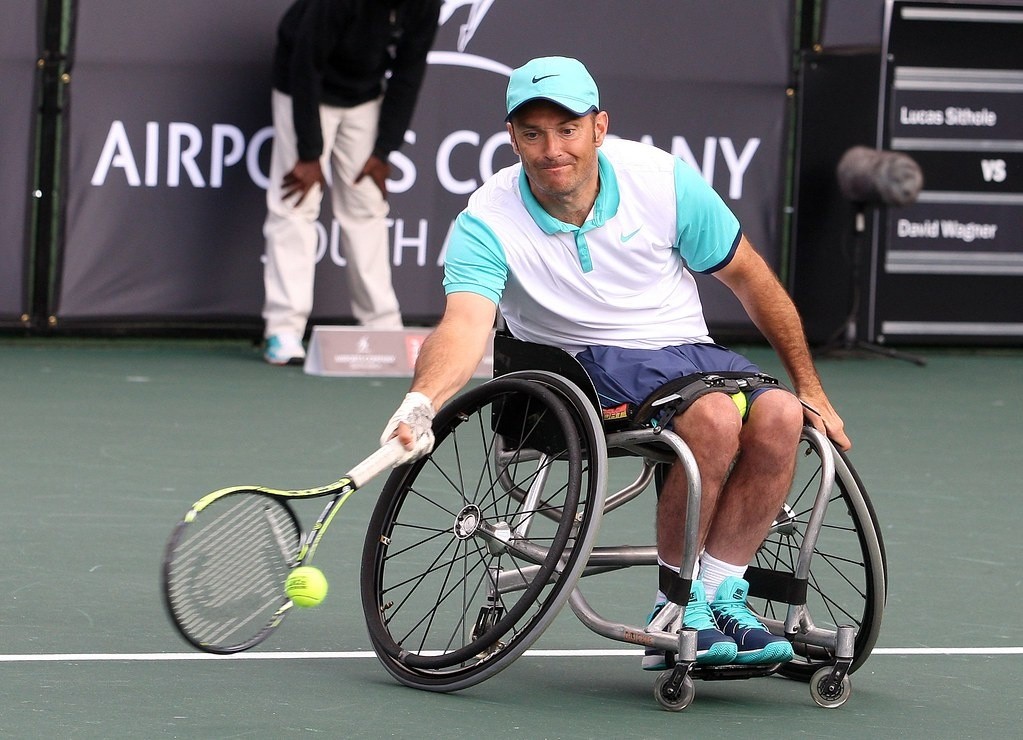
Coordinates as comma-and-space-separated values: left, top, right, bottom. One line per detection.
505, 56, 600, 121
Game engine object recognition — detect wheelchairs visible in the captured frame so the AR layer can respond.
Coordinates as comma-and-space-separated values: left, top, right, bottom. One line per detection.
357, 328, 889, 713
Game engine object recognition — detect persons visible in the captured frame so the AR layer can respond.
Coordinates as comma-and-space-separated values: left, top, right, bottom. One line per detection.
378, 55, 852, 671
261, 0, 443, 365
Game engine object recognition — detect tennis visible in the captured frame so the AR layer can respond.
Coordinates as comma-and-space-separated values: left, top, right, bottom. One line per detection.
284, 566, 328, 608
731, 391, 747, 416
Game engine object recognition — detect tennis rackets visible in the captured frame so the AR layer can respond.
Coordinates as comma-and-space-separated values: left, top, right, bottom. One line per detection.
161, 430, 418, 655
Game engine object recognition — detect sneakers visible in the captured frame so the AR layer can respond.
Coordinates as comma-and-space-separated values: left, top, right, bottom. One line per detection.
262, 335, 305, 365
641, 580, 737, 671
708, 575, 794, 664
